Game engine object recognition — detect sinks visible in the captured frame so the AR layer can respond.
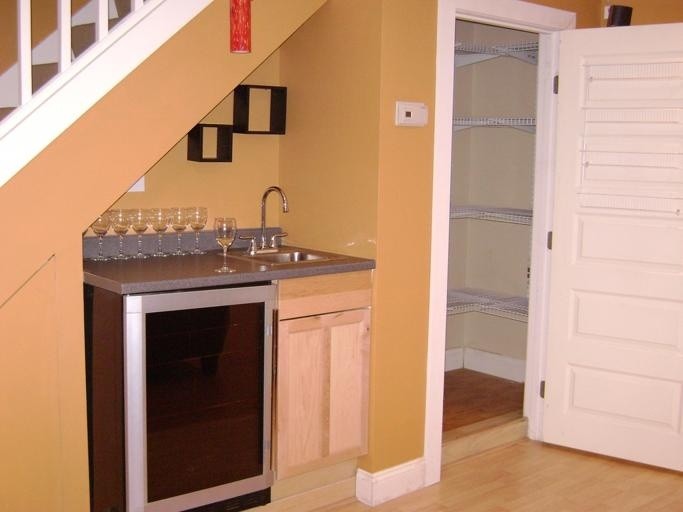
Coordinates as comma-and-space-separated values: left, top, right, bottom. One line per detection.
218, 250, 350, 265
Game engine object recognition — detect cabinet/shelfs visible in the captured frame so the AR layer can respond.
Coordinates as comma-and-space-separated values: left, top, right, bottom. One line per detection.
274, 269, 376, 478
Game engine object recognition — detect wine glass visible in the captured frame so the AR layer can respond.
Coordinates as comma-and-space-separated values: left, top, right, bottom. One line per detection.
214, 218, 236, 273
132, 209, 151, 260
171, 207, 190, 255
113, 209, 132, 260
151, 208, 171, 257
91, 210, 111, 262
189, 207, 208, 254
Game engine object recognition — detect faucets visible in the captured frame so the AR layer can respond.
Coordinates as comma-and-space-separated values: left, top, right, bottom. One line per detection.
239, 186, 288, 256
